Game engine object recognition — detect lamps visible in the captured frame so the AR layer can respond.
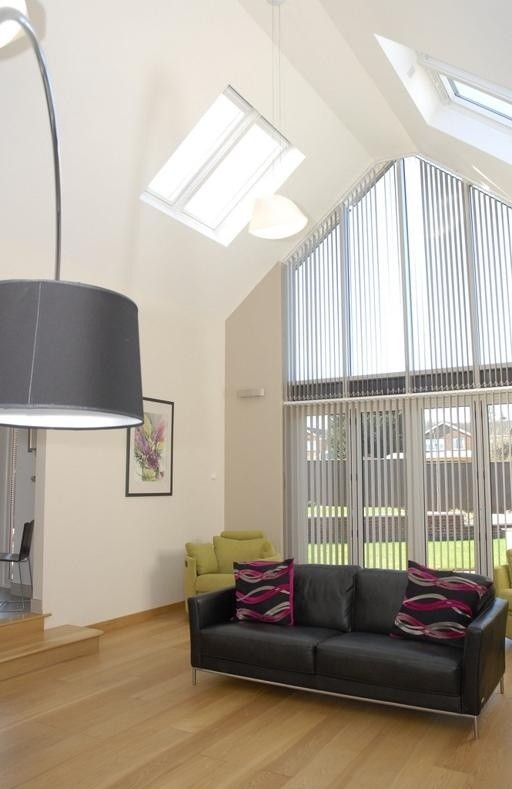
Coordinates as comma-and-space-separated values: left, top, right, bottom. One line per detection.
1, 0, 143, 430
248, 0, 308, 240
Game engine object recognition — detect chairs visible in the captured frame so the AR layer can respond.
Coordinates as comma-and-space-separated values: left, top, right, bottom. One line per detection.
493, 566, 512, 639
185, 530, 283, 614
0, 520, 34, 610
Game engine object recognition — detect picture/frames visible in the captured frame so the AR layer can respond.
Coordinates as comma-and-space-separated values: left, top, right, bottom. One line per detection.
28, 429, 37, 452
126, 397, 174, 496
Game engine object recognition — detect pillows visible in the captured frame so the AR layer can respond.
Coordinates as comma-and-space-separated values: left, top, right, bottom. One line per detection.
357, 566, 408, 635
294, 564, 358, 634
389, 560, 492, 647
222, 530, 262, 540
233, 559, 295, 626
210, 536, 265, 573
506, 548, 512, 588
184, 543, 216, 575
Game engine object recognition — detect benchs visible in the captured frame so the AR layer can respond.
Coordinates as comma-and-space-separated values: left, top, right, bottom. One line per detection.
179, 564, 509, 741
